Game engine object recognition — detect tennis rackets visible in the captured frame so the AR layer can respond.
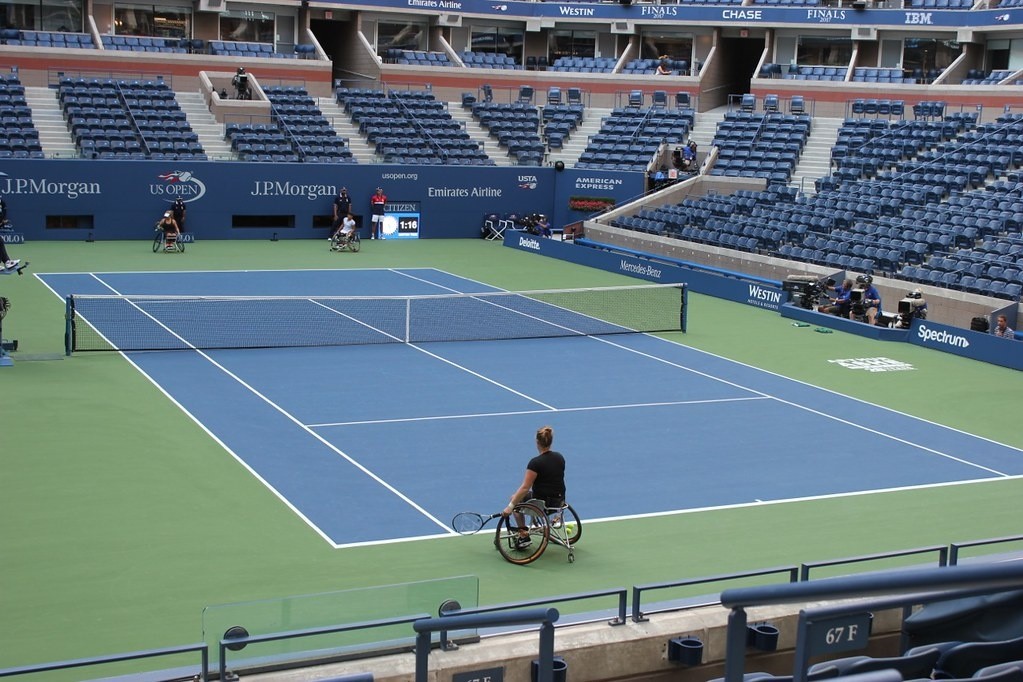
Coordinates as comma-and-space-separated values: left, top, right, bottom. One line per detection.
452, 511, 502, 536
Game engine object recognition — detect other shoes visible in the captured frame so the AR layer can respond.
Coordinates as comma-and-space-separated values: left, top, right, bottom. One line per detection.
370, 233, 375, 240
379, 234, 385, 240
0, 266, 5, 271
5, 260, 21, 268
516, 536, 532, 547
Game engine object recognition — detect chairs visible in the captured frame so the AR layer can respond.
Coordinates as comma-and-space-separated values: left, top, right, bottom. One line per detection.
709, 636, 1023, 682
0, 0, 1023, 301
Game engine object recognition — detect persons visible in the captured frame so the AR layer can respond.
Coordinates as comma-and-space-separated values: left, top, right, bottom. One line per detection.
157, 196, 186, 247
655, 61, 671, 75
502, 426, 566, 548
231, 67, 253, 99
328, 141, 700, 250
819, 274, 928, 328
994, 315, 1015, 339
0, 195, 21, 271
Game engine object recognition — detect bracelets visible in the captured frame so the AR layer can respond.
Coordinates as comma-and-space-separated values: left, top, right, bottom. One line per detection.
509, 502, 515, 509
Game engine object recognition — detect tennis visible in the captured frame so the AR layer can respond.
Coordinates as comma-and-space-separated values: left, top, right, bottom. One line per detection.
566, 527, 571, 534
567, 524, 574, 529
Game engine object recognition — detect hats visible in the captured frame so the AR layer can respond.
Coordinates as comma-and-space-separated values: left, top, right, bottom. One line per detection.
164, 212, 170, 217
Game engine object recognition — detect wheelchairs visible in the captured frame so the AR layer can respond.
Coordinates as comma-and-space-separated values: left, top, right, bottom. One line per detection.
330, 230, 361, 252
494, 499, 582, 565
153, 231, 185, 254
